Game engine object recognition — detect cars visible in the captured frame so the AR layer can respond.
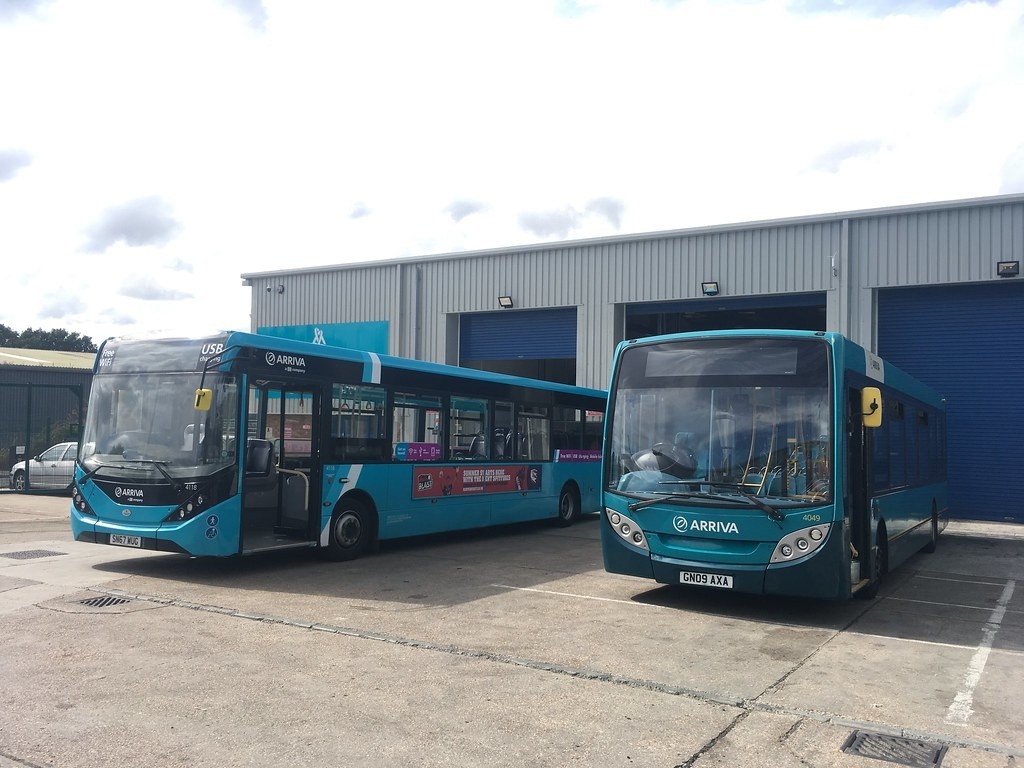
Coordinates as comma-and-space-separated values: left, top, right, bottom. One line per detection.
9, 442, 78, 494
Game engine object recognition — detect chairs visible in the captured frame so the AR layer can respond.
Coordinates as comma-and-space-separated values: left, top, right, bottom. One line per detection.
469, 427, 570, 459
246, 438, 275, 477
741, 435, 827, 497
674, 432, 709, 478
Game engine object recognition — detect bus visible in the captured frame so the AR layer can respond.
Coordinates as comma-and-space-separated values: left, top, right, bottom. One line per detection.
600, 329, 948, 599
71, 331, 608, 562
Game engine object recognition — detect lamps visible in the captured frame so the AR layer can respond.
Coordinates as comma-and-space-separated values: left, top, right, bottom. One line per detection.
498, 296, 513, 309
997, 261, 1019, 278
701, 282, 719, 296
